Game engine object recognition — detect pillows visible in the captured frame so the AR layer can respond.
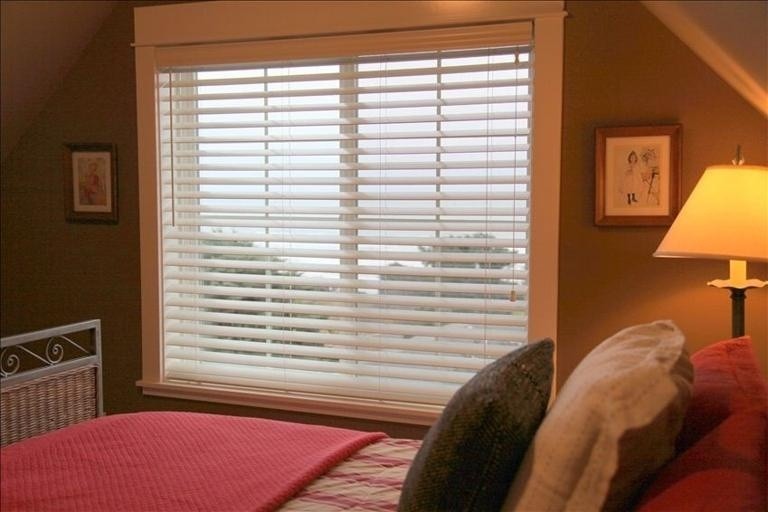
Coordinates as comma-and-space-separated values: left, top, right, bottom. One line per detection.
505, 318, 692, 511
621, 332, 766, 511
393, 338, 555, 511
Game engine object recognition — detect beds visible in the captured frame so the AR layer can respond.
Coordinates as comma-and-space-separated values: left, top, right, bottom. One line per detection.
1, 316, 768, 510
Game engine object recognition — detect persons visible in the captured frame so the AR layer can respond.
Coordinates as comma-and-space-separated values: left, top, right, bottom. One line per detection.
619, 150, 645, 204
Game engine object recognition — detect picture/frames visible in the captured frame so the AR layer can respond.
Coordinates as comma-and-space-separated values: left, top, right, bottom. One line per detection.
593, 123, 681, 228
62, 142, 119, 225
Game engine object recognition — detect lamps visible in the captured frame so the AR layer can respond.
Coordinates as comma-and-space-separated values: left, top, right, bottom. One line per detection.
646, 144, 768, 341
